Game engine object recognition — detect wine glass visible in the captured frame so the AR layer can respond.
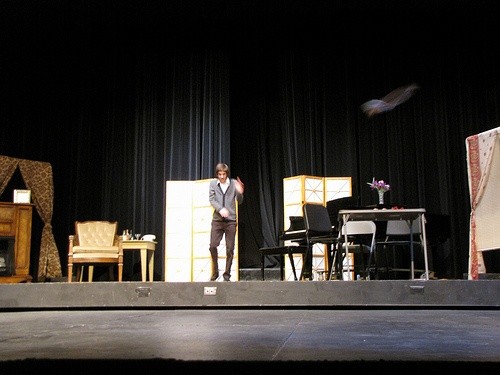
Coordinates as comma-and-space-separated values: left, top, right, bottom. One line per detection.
135, 234, 141, 240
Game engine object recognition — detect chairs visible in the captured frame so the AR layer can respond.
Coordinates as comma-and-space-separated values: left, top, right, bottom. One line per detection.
328, 220, 378, 282
66, 220, 123, 282
298, 202, 351, 281
376, 215, 422, 279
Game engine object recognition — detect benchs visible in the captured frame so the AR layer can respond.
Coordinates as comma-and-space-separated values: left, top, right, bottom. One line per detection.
257, 245, 306, 282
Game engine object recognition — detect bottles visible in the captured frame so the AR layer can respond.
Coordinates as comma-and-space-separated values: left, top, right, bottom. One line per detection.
123, 229, 132, 240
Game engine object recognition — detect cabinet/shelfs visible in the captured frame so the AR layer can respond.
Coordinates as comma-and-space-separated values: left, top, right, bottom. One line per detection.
0, 201, 37, 283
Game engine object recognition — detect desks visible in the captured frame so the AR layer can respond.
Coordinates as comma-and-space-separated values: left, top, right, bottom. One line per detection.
122, 239, 158, 282
338, 207, 430, 279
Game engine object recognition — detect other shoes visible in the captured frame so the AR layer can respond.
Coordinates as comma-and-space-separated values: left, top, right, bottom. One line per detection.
210, 272, 219, 281
224, 275, 230, 281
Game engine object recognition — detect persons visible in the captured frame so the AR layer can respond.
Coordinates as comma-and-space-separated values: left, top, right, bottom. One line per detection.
209, 163, 244, 281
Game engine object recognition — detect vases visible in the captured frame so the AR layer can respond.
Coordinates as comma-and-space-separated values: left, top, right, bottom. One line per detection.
378, 190, 386, 209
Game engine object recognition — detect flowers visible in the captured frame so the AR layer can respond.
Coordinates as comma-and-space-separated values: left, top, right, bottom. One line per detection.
366, 176, 392, 192
